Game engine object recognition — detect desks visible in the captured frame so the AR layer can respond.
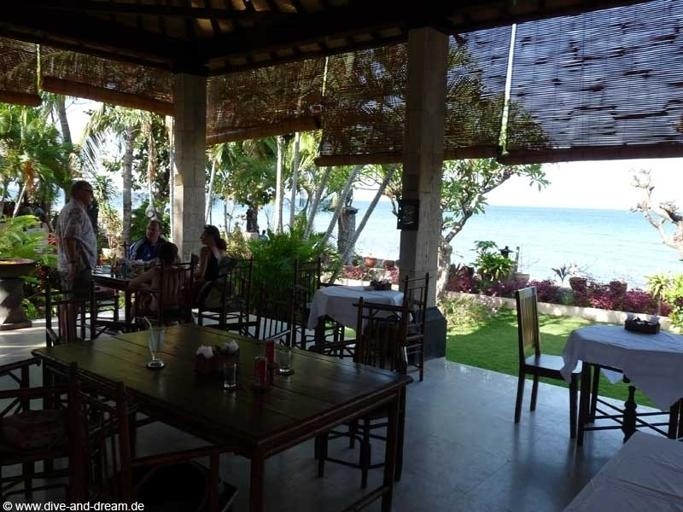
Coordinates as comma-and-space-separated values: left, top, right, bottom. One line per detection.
562, 326, 682, 512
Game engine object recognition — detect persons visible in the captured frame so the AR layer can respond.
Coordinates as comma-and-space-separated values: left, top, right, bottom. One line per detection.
114, 240, 178, 324
32, 202, 45, 218
56, 180, 97, 348
21, 203, 33, 216
177, 223, 224, 323
124, 220, 174, 267
6, 202, 19, 216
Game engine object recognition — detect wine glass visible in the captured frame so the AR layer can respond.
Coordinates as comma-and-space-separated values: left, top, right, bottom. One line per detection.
147, 326, 165, 369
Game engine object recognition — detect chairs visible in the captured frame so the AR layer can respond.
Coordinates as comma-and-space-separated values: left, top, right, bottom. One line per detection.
513, 285, 582, 438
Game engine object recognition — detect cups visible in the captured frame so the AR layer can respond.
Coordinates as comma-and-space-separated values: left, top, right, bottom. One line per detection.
223, 361, 237, 388
279, 350, 291, 372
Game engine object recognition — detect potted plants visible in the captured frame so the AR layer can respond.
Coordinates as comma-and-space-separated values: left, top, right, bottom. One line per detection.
226, 209, 352, 323
